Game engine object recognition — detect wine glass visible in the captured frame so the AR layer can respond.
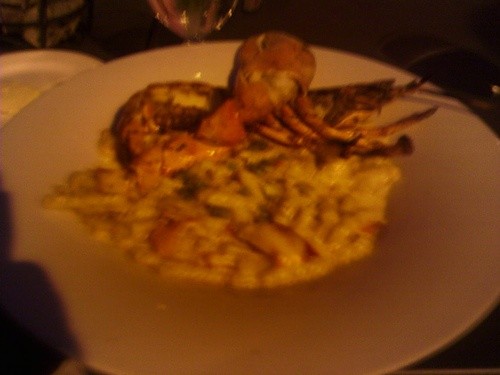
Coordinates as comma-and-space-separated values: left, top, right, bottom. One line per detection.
149, 1, 237, 42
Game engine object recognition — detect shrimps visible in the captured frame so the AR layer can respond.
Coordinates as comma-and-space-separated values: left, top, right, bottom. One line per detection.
109, 76, 428, 175
194, 33, 440, 146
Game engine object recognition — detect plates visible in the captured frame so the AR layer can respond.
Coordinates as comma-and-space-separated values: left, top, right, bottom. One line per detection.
1, 42, 499, 373
0, 49, 104, 121
